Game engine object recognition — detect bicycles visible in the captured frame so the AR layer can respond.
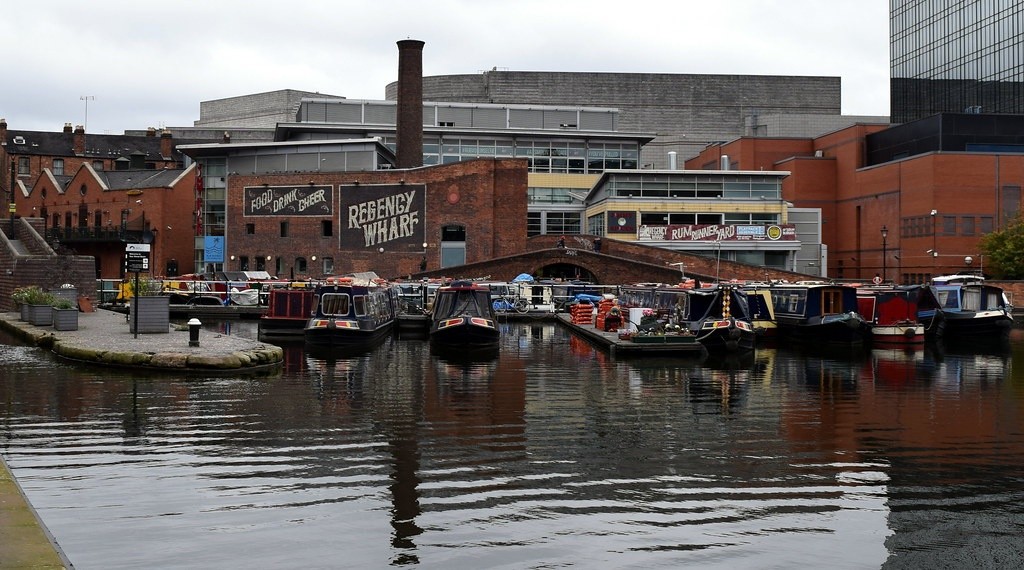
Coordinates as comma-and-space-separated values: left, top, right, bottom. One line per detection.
493, 292, 530, 314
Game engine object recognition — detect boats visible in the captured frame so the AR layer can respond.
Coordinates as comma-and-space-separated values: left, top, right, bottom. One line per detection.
303, 276, 404, 352
428, 279, 501, 355
743, 278, 873, 346
927, 283, 1014, 333
740, 288, 779, 339
839, 282, 925, 344
681, 283, 756, 342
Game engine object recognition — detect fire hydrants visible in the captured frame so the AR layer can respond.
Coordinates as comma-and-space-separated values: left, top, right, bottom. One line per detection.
187, 318, 202, 346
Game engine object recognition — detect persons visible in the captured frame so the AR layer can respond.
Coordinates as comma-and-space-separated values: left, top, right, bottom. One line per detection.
557, 236, 565, 248
593, 236, 602, 252
420, 257, 427, 272
